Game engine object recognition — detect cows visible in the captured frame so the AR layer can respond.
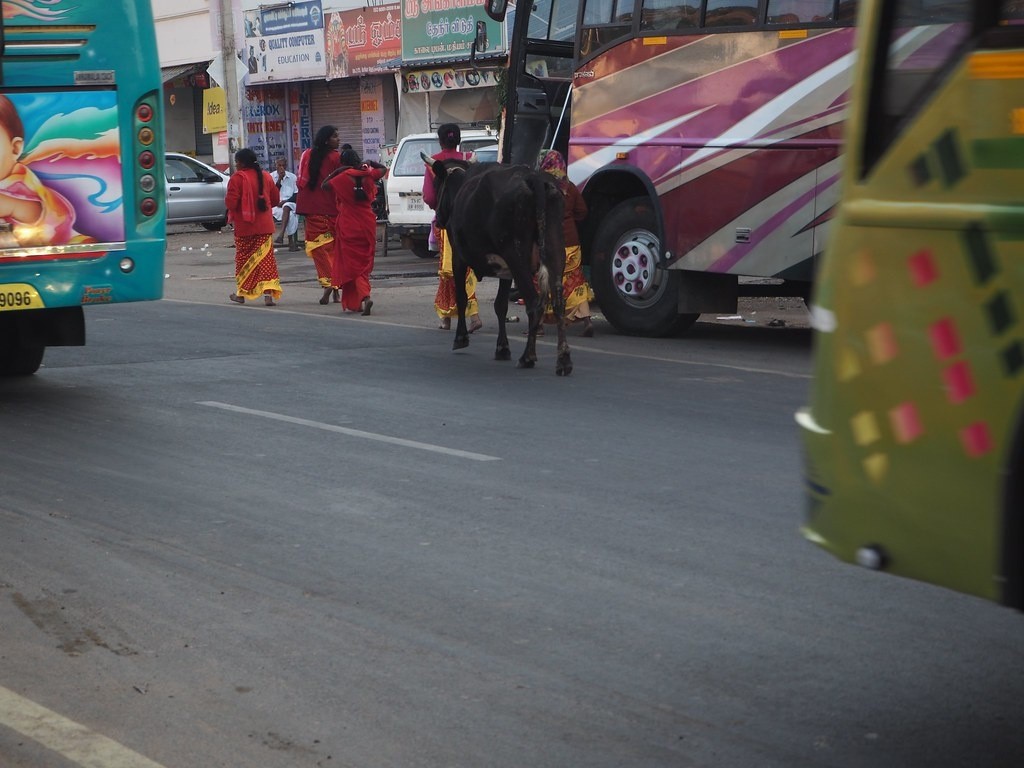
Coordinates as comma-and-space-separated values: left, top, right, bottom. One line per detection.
420, 150, 573, 376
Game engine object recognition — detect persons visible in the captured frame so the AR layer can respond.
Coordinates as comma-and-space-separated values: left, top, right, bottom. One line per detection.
267, 155, 300, 252
522, 149, 597, 339
424, 124, 484, 335
226, 148, 282, 306
320, 149, 389, 317
297, 124, 344, 304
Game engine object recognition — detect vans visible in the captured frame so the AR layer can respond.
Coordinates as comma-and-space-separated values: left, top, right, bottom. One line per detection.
387, 127, 500, 257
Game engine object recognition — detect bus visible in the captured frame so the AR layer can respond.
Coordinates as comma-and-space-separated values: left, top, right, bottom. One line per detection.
469, 0, 975, 340
793, 0, 1024, 617
0, 0, 169, 379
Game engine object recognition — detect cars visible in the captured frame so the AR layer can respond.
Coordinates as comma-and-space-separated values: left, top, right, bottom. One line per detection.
472, 145, 499, 167
164, 152, 230, 230
